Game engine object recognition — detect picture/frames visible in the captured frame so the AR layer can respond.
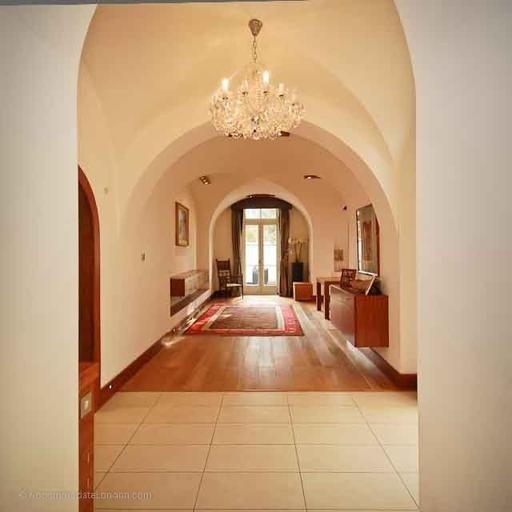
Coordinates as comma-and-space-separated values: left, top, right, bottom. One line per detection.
355, 204, 380, 278
174, 201, 190, 248
364, 275, 376, 295
340, 268, 356, 287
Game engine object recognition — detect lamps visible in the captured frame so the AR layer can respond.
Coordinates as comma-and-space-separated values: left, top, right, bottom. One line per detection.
208, 18, 306, 143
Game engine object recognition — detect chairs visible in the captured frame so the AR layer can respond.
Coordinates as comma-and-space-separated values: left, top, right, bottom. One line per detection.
216, 258, 244, 300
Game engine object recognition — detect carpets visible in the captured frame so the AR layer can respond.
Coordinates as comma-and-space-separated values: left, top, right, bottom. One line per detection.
177, 303, 304, 336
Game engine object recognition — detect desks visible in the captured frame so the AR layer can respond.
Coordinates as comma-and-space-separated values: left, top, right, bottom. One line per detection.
316, 277, 343, 320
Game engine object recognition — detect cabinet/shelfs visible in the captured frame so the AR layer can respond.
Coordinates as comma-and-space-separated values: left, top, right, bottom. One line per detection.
292, 282, 313, 301
329, 284, 389, 348
79, 360, 101, 512
